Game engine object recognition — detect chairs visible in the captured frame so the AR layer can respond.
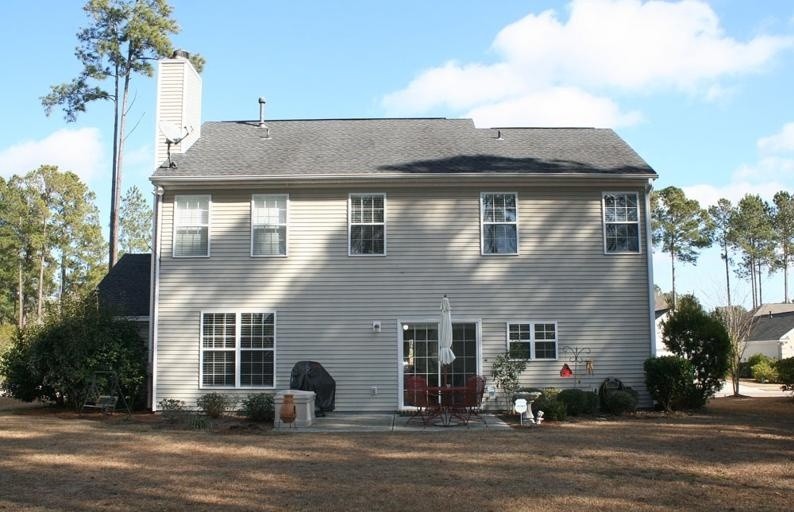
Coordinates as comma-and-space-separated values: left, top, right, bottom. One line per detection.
452, 373, 490, 429
404, 372, 432, 427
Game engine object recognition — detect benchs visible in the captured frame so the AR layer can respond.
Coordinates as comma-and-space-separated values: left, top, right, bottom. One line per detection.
78, 395, 119, 419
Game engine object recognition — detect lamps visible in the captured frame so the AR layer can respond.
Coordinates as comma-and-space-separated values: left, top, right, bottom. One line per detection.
558, 344, 596, 389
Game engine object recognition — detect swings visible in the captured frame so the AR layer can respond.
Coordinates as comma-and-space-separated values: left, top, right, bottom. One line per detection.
84, 374, 118, 410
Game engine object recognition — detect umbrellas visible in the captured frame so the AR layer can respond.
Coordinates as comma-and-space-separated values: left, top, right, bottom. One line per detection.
437, 292, 457, 388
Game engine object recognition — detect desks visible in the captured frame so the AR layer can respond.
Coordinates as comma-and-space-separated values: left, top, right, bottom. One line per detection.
419, 385, 470, 427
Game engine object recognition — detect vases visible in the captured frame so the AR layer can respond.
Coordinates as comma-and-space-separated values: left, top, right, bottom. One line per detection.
277, 393, 297, 422
